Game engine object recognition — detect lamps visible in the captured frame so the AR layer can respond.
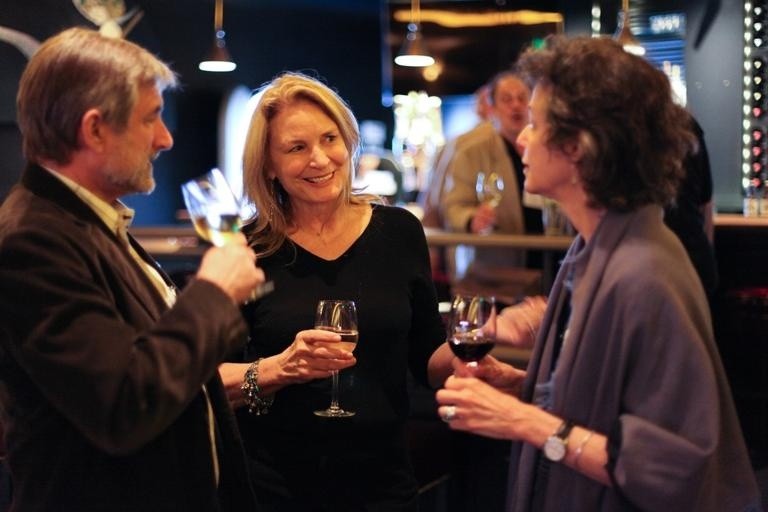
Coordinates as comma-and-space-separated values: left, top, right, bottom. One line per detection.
393, 3, 435, 67
198, 1, 237, 73
615, 2, 646, 58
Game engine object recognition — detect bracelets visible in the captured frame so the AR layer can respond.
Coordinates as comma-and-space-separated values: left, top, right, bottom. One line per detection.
572, 427, 598, 477
237, 356, 278, 416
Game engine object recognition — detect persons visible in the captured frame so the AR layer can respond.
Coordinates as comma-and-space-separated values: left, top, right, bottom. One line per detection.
674, 109, 729, 305
454, 35, 766, 512
442, 73, 561, 301
418, 85, 492, 283
0, 28, 266, 511
206, 74, 550, 512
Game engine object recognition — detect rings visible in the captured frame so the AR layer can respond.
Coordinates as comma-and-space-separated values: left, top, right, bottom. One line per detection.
443, 406, 459, 423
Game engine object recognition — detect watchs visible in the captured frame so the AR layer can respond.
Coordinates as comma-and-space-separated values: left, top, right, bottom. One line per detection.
543, 418, 577, 464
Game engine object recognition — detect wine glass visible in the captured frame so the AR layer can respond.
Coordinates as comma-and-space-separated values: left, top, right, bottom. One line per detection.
475, 172, 503, 240
313, 299, 357, 422
450, 292, 496, 376
182, 167, 274, 308
